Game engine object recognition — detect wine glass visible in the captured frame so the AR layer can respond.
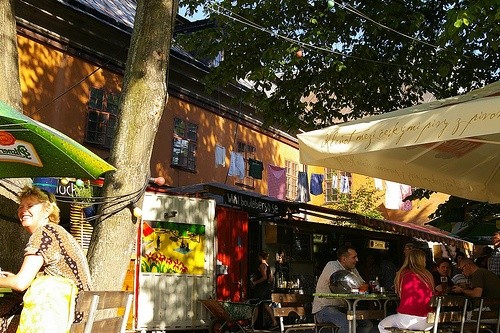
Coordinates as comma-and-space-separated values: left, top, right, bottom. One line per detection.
370, 280, 378, 293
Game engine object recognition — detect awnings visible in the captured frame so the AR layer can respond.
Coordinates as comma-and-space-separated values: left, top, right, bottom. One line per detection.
352, 202, 465, 250
296, 80, 500, 294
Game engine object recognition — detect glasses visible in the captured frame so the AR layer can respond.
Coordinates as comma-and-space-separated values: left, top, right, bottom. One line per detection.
460, 262, 471, 272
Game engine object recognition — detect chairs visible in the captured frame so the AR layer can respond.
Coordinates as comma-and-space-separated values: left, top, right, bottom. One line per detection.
70, 291, 134, 333
393, 296, 468, 333
196, 293, 359, 333
471, 298, 500, 333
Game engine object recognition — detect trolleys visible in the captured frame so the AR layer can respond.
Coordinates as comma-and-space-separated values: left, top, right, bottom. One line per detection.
200, 297, 273, 333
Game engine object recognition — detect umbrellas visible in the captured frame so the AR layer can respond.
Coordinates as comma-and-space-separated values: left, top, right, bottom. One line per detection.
0, 100, 118, 182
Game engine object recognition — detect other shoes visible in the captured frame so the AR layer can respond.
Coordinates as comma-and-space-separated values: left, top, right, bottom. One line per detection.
243, 325, 254, 330
268, 325, 279, 330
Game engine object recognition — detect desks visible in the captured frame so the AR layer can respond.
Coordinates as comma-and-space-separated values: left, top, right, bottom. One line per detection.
312, 293, 387, 333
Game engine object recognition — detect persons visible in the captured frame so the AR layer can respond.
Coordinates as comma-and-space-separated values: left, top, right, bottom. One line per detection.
377, 248, 433, 333
312, 243, 368, 333
248, 251, 280, 332
487, 231, 500, 275
451, 258, 500, 319
0, 185, 94, 333
403, 243, 416, 257
456, 252, 465, 265
432, 258, 465, 290
